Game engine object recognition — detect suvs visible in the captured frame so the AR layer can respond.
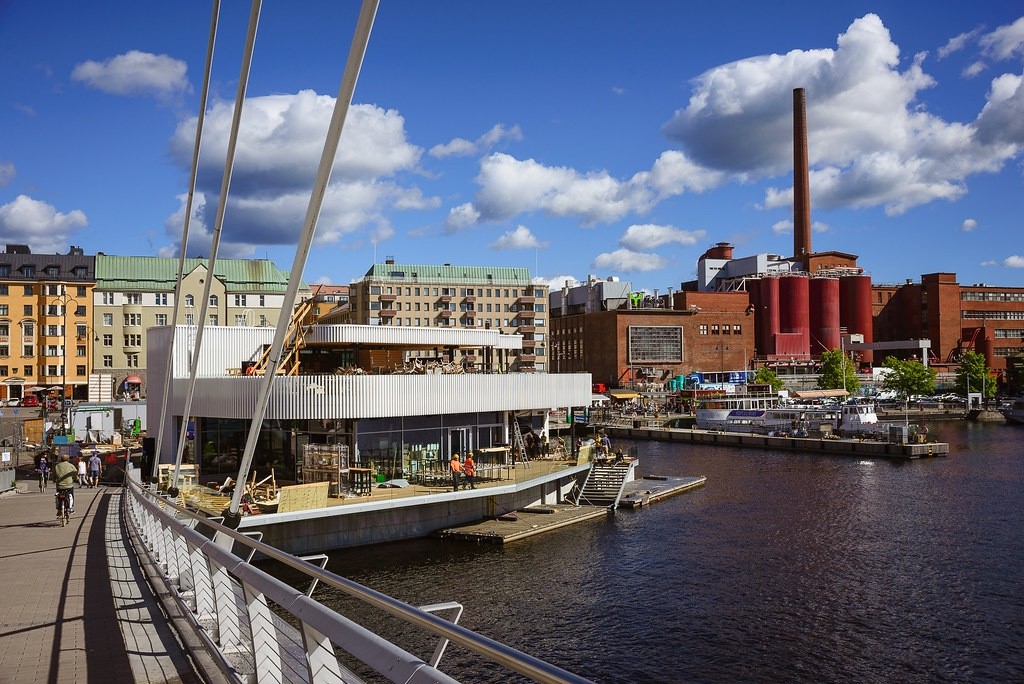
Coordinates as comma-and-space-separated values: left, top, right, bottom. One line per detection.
593, 383, 606, 394
23, 395, 39, 407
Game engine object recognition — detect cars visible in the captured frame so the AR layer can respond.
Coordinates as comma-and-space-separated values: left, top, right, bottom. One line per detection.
7, 398, 21, 407
0, 399, 3, 408
909, 392, 968, 403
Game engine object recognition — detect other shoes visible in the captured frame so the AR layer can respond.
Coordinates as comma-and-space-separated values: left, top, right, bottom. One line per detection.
70, 510, 75, 514
90, 483, 93, 488
56, 511, 62, 516
87, 483, 89, 488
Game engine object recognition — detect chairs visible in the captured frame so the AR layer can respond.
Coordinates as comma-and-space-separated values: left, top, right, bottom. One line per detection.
393, 356, 467, 374
337, 365, 367, 376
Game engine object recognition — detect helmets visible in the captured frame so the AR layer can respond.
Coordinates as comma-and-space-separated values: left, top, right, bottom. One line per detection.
468, 453, 474, 457
453, 454, 459, 459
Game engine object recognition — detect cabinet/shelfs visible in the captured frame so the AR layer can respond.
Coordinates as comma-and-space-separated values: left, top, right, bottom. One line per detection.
350, 467, 371, 496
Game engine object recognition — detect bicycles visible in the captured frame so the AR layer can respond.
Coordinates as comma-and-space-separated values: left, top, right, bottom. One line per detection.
36, 469, 49, 493
55, 488, 70, 527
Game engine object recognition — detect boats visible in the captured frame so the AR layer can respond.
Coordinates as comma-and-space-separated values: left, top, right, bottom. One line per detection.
696, 348, 918, 439
997, 396, 1024, 423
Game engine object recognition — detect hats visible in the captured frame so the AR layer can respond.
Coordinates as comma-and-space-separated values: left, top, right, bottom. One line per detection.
61, 454, 69, 462
93, 451, 97, 455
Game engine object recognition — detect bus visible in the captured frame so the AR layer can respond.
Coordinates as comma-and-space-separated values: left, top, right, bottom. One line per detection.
686, 368, 770, 393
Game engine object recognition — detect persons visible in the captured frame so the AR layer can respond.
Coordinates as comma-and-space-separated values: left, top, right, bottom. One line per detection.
591, 432, 624, 468
575, 437, 583, 459
130, 390, 140, 401
35, 451, 102, 516
526, 430, 567, 459
344, 363, 363, 375
592, 400, 691, 418
246, 363, 255, 376
450, 452, 477, 491
